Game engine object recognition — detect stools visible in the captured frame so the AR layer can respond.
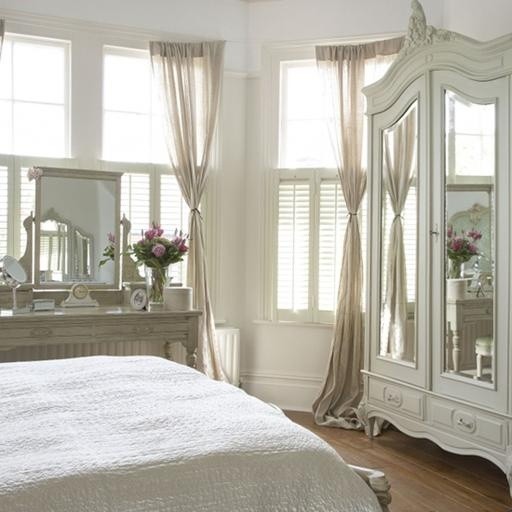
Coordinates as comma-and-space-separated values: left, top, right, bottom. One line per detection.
473, 335, 492, 377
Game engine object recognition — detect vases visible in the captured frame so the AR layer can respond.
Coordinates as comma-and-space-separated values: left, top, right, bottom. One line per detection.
145, 267, 167, 312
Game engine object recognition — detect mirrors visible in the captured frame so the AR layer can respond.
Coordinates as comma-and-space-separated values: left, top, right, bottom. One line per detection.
1, 165, 147, 308
40, 207, 94, 285
448, 204, 493, 292
0, 254, 31, 315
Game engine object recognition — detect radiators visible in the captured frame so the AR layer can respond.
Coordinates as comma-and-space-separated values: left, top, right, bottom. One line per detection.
13, 343, 151, 360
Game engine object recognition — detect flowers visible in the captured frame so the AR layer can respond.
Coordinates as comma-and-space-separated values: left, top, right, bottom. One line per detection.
118, 222, 194, 303
447, 223, 483, 279
98, 231, 114, 271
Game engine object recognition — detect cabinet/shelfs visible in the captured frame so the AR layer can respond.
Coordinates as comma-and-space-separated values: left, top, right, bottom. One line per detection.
355, 0, 512, 499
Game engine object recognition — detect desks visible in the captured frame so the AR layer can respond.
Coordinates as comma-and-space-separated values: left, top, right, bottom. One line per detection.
0, 302, 203, 370
446, 295, 493, 372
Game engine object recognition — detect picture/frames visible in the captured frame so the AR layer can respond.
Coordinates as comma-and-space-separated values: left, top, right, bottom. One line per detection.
129, 289, 147, 310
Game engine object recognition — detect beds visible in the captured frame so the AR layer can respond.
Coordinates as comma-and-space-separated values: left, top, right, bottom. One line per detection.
0, 355, 392, 512
